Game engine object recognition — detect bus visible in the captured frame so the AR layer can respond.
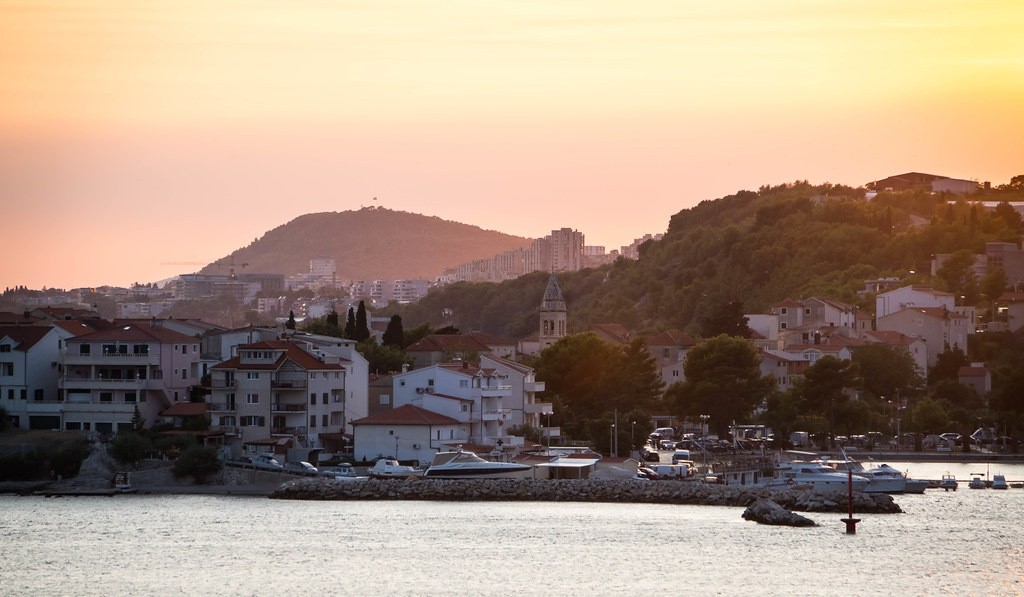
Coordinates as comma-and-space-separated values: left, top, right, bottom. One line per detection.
648, 465, 687, 479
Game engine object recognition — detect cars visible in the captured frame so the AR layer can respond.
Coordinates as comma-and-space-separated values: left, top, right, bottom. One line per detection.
637, 467, 659, 478
640, 428, 729, 473
255, 455, 283, 469
285, 461, 318, 476
792, 427, 1024, 448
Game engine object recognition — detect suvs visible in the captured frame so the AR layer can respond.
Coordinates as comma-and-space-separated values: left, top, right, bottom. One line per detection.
323, 463, 356, 477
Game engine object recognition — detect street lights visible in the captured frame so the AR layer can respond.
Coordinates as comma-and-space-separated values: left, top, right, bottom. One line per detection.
699, 415, 710, 481
609, 424, 616, 456
632, 421, 637, 457
543, 411, 553, 462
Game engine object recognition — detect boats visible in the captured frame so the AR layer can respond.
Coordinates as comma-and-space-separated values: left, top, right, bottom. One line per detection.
423, 451, 532, 478
367, 459, 424, 477
992, 475, 1008, 489
940, 474, 957, 491
968, 473, 986, 488
784, 457, 928, 493
